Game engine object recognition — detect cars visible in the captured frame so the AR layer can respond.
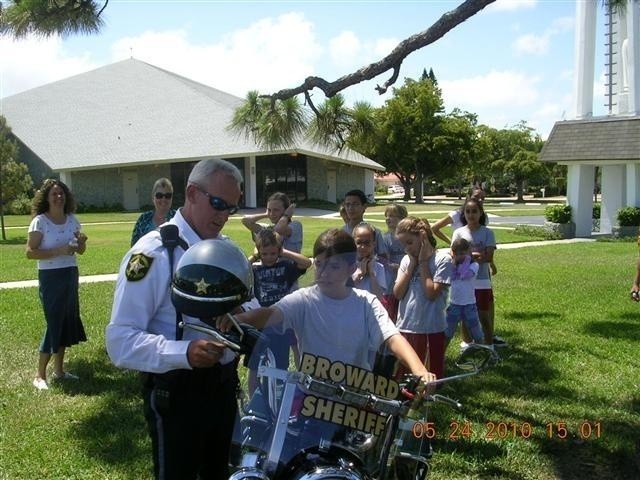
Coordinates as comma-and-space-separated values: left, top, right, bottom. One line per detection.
388, 184, 404, 194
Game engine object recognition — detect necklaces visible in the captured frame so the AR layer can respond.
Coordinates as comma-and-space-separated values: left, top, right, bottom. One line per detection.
156, 210, 167, 220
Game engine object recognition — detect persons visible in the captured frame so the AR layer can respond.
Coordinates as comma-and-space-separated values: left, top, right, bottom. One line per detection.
340, 189, 497, 394
26, 176, 90, 391
628, 259, 639, 304
243, 192, 312, 400
216, 231, 438, 390
131, 174, 177, 247
108, 156, 268, 475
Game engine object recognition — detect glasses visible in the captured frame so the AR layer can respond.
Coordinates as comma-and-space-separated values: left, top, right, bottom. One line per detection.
193, 184, 240, 216
155, 192, 172, 200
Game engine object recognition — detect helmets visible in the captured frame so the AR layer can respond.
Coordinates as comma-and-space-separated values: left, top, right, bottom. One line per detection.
170, 238, 256, 319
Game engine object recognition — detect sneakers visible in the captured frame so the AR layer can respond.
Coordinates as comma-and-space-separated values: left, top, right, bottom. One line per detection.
51, 371, 80, 381
32, 377, 52, 391
460, 335, 508, 353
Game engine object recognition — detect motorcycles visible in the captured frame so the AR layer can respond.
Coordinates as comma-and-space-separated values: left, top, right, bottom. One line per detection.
178, 306, 501, 479
